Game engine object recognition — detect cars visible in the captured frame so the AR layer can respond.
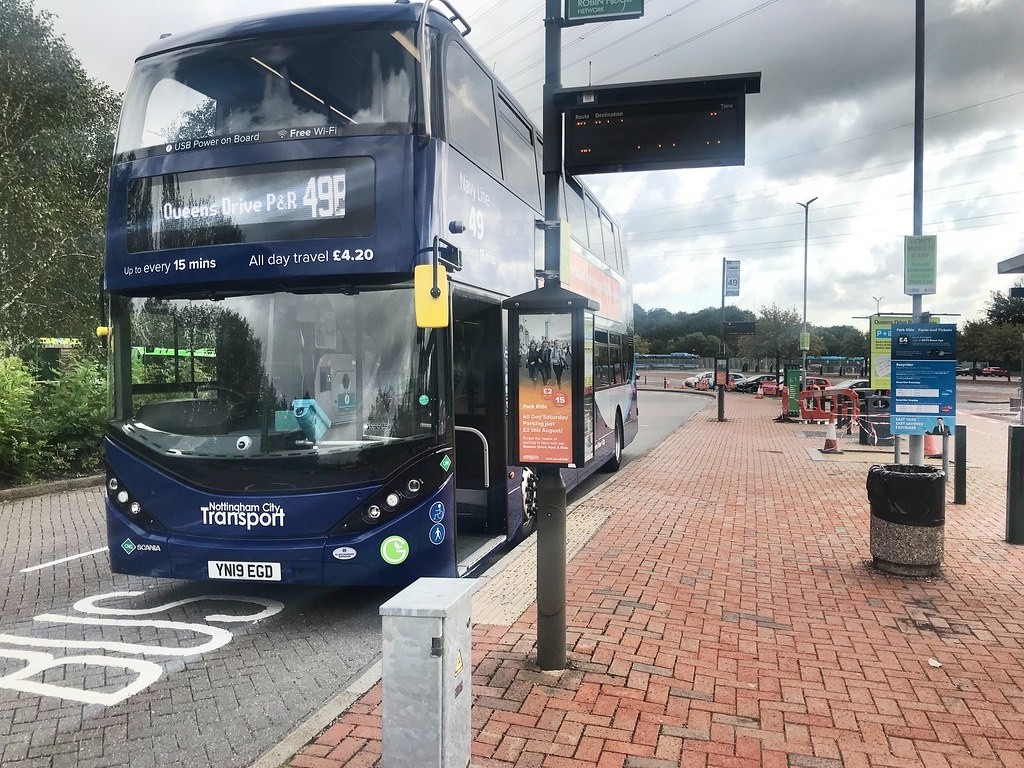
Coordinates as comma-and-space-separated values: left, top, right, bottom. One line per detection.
769, 376, 830, 395
734, 374, 777, 393
956, 366, 982, 376
684, 372, 715, 388
708, 373, 747, 390
824, 380, 877, 402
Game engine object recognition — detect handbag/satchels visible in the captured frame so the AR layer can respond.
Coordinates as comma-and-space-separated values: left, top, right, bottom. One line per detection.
559, 357, 570, 370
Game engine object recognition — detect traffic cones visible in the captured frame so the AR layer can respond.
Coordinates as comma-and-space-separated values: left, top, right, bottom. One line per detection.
667, 379, 670, 388
680, 380, 687, 389
755, 385, 765, 400
818, 413, 843, 453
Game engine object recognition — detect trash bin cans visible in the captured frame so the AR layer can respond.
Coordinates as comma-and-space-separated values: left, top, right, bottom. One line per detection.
862, 396, 893, 446
868, 465, 945, 579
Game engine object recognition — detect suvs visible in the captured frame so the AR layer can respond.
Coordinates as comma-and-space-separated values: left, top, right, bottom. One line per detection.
982, 367, 1012, 377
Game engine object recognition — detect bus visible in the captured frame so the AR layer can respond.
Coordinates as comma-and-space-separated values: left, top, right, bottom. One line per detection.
799, 357, 864, 373
99, 1, 637, 597
635, 353, 700, 370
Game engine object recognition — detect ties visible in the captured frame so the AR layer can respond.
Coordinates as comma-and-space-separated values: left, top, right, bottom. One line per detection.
940, 426, 943, 433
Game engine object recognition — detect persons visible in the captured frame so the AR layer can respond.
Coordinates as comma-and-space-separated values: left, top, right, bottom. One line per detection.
527, 340, 571, 390
922, 417, 952, 436
